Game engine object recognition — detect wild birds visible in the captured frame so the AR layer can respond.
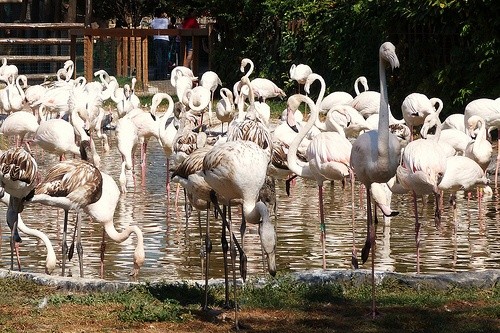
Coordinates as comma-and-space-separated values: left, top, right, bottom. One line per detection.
0, 41, 500, 333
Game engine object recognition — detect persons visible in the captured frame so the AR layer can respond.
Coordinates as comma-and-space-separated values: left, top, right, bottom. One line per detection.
150, 8, 201, 79
